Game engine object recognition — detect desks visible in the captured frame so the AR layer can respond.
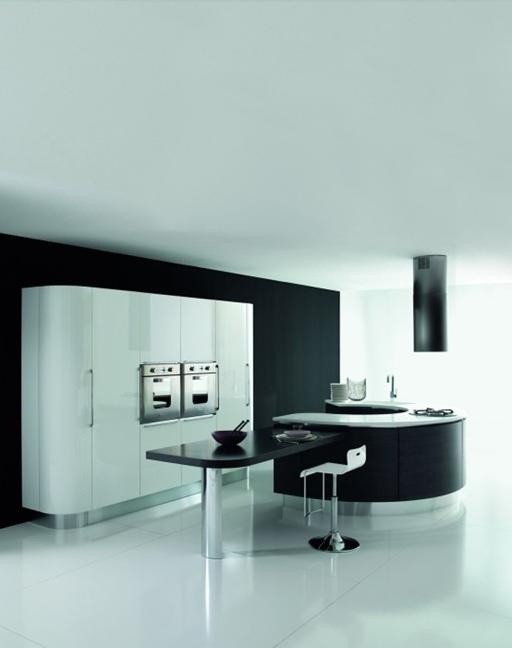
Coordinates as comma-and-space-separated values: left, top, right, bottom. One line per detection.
146, 428, 342, 560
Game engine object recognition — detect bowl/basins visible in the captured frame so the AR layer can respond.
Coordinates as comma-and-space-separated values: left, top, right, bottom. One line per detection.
284, 430, 313, 438
211, 431, 248, 445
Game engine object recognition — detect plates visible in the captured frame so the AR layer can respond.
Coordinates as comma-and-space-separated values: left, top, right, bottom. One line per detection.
329, 382, 349, 401
275, 432, 318, 443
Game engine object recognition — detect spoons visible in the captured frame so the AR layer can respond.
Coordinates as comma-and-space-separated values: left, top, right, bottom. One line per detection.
277, 438, 296, 443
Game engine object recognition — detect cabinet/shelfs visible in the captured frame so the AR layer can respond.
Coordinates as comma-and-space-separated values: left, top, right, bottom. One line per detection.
216, 299, 254, 475
140, 291, 216, 363
19, 286, 139, 517
140, 414, 218, 496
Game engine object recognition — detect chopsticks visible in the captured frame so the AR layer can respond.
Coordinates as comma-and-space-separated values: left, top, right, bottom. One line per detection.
234, 419, 250, 430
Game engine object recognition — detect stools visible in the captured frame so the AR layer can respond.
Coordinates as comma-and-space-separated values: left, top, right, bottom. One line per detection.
299, 444, 366, 553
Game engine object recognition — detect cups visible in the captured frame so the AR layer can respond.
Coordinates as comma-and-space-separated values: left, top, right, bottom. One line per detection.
347, 375, 367, 402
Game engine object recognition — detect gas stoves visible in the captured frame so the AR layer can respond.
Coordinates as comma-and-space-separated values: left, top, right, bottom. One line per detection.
409, 405, 457, 417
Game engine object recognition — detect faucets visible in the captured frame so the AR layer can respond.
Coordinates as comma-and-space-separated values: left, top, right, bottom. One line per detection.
387, 375, 396, 398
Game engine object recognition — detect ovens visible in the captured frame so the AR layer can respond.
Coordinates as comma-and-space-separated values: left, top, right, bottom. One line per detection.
140, 362, 218, 424
